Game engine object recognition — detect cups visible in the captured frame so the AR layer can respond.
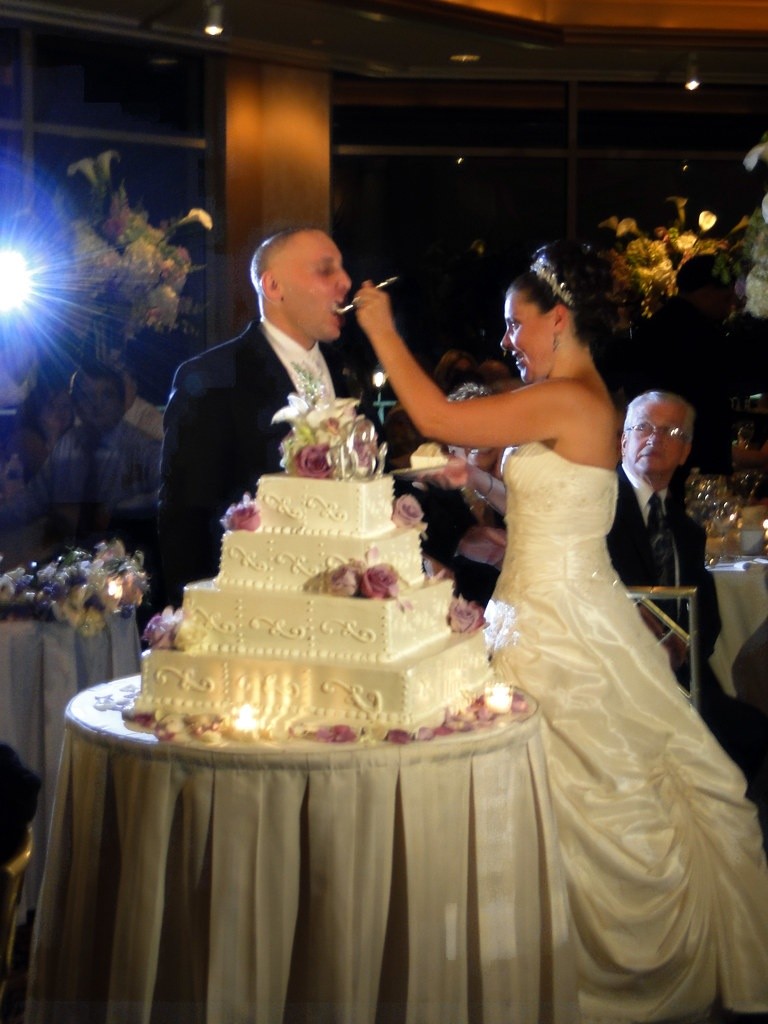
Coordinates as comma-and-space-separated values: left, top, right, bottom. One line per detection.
738, 530, 765, 555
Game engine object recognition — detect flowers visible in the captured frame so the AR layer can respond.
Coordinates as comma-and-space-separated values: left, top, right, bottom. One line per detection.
579, 195, 750, 338
714, 130, 767, 346
62, 145, 216, 345
2, 534, 152, 640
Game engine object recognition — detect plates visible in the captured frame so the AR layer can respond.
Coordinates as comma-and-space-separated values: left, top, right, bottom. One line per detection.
392, 461, 449, 483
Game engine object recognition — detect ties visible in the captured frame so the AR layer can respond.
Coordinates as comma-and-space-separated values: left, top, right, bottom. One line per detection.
73, 445, 99, 552
646, 493, 678, 632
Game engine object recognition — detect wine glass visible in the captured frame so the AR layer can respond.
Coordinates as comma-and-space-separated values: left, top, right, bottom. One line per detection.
683, 469, 762, 567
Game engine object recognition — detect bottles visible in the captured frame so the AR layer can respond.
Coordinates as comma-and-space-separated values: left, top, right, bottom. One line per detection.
685, 467, 701, 489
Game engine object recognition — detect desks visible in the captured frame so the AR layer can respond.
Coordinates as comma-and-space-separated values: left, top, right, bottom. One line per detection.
21, 674, 583, 1024
704, 519, 768, 697
0, 604, 142, 924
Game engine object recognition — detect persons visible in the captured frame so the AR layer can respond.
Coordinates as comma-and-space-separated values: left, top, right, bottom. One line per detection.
351, 235, 768, 1023
152, 224, 384, 620
1, 211, 767, 799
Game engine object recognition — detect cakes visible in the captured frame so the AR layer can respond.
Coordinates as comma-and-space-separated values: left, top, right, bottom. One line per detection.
409, 442, 449, 470
130, 391, 498, 738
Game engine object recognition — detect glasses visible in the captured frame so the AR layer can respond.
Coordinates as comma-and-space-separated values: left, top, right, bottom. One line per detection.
626, 421, 690, 442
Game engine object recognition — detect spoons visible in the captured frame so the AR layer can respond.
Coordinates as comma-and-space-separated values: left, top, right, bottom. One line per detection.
339, 277, 399, 314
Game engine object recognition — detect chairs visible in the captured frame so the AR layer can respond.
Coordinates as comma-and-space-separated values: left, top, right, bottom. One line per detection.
625, 587, 702, 715
0, 811, 42, 1007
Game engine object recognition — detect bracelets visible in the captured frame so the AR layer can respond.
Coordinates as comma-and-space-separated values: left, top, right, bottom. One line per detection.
474, 471, 494, 500
